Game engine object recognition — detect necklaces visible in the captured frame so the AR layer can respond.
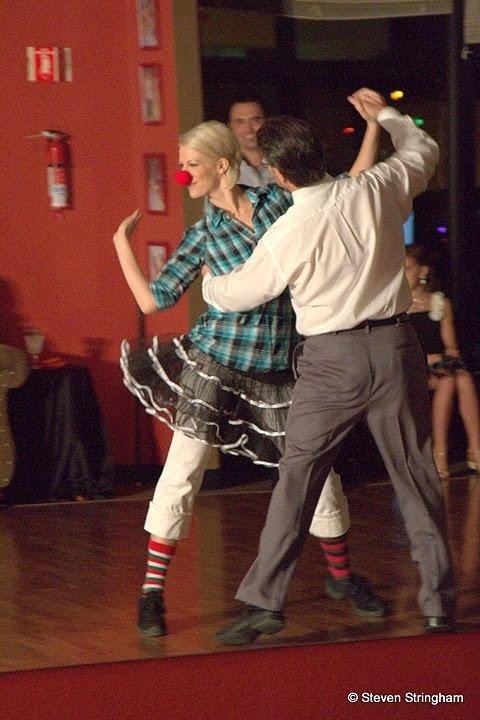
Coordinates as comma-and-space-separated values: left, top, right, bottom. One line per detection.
407, 289, 428, 310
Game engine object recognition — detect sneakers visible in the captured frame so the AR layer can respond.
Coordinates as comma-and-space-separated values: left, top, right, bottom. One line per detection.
138, 591, 167, 637
325, 572, 386, 618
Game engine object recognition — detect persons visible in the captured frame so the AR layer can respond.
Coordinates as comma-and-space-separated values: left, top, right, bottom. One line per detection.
111, 93, 387, 638
223, 98, 286, 489
400, 244, 480, 478
202, 88, 459, 647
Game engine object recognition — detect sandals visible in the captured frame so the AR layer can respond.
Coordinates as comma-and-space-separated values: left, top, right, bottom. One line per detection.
432, 452, 450, 480
466, 450, 480, 474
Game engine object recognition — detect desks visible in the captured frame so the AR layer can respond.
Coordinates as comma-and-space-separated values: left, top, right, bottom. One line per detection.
8, 362, 122, 500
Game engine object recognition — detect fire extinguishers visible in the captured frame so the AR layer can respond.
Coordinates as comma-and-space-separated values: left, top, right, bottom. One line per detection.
40, 131, 71, 210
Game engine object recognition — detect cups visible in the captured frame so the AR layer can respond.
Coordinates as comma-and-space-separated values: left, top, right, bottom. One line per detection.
23, 334, 46, 363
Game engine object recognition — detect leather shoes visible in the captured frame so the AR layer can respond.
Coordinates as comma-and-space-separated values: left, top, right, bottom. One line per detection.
424, 615, 457, 632
215, 606, 285, 646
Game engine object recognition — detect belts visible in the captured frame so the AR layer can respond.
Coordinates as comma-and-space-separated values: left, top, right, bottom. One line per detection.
353, 312, 410, 331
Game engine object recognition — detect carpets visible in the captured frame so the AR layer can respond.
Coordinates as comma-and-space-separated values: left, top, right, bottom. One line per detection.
0, 475, 480, 672
